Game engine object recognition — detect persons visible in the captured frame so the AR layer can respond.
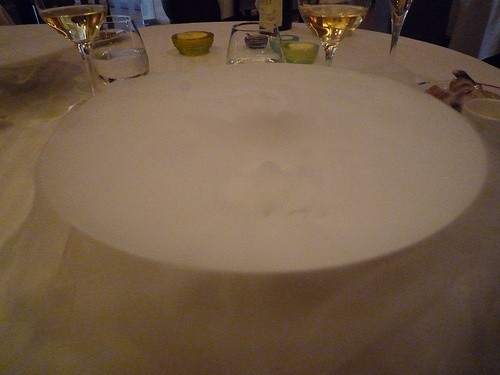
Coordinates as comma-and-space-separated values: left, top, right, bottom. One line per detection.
71, 0, 500, 73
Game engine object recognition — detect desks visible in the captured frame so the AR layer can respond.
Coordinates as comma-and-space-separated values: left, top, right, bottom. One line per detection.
0, 21, 500, 375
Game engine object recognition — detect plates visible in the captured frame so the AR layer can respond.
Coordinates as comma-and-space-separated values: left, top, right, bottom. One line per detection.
417, 80, 500, 117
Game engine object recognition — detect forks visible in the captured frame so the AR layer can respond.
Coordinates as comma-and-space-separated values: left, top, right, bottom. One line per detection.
453, 70, 500, 89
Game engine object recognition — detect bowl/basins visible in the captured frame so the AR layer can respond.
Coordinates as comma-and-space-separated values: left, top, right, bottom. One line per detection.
283, 41, 319, 64
270, 34, 298, 53
171, 31, 215, 56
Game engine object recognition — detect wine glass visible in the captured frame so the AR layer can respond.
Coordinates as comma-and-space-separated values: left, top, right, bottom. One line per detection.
35, 0, 107, 112
373, 0, 415, 73
298, 0, 371, 67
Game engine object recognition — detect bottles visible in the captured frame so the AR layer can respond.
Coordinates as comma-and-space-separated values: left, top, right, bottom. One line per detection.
258, 0, 292, 30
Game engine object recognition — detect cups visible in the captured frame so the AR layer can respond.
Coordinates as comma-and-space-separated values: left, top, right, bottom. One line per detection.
227, 22, 285, 63
92, 15, 149, 85
463, 98, 500, 148
